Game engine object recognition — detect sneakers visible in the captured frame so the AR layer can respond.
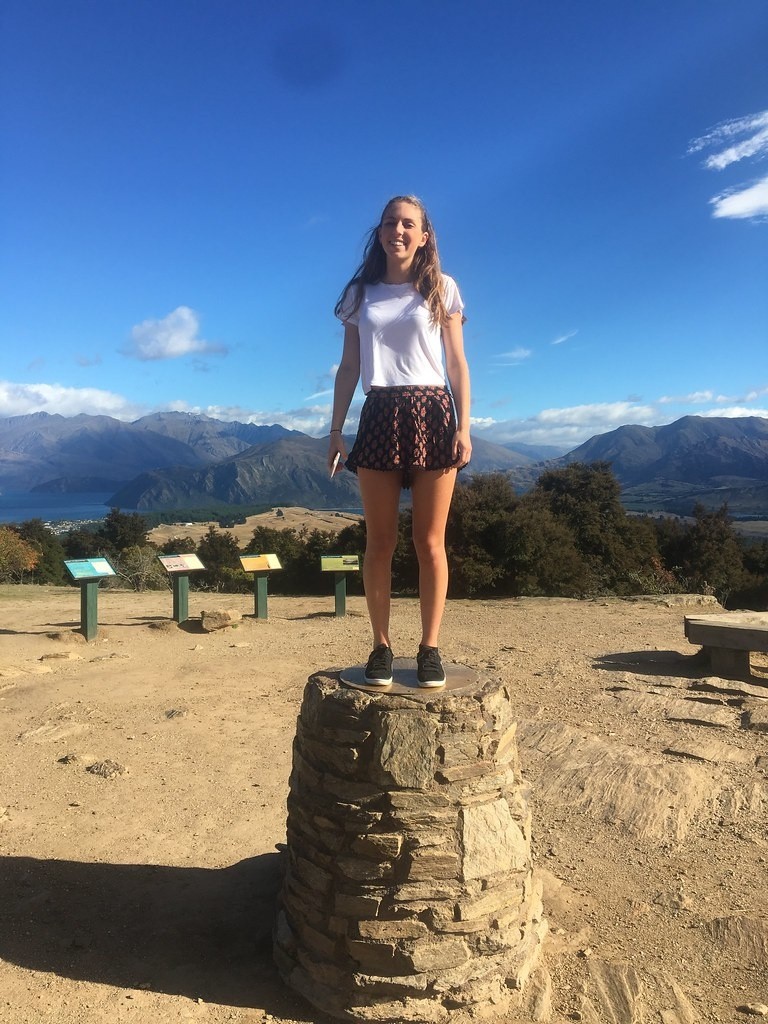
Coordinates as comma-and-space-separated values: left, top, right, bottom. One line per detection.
364, 644, 394, 686
417, 644, 446, 687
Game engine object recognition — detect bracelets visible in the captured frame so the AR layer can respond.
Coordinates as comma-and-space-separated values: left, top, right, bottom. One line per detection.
329, 429, 343, 435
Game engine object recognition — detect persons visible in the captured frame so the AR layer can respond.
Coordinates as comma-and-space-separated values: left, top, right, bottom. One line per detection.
329, 196, 472, 687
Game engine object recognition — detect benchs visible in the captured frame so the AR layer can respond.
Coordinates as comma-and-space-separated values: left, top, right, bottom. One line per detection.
684, 611, 768, 676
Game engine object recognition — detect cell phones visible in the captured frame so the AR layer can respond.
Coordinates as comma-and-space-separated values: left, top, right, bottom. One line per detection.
328, 452, 341, 479
